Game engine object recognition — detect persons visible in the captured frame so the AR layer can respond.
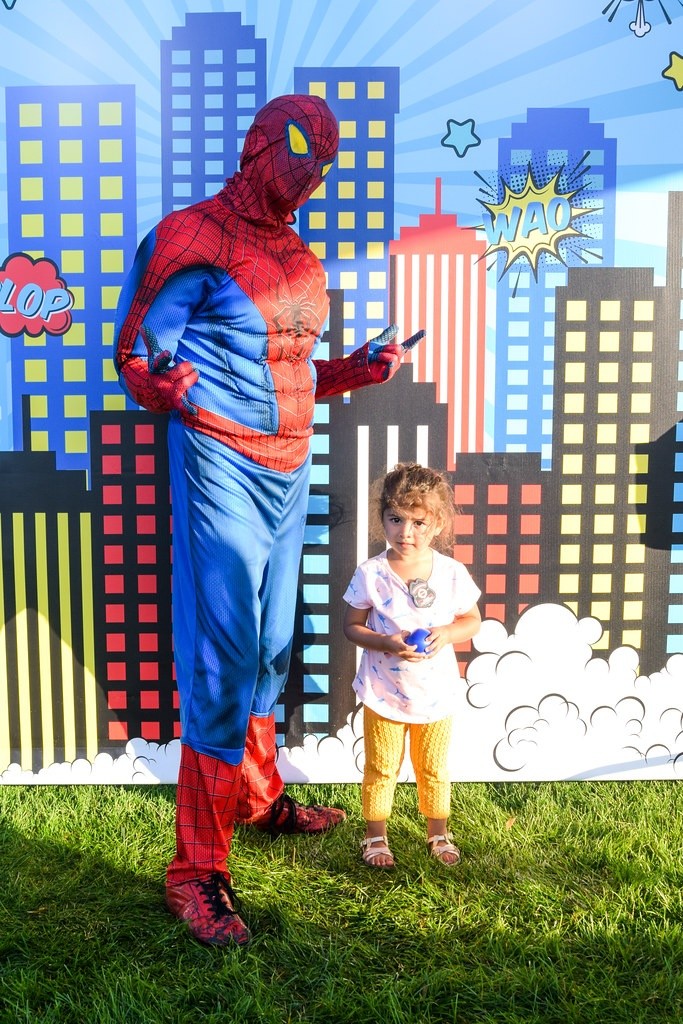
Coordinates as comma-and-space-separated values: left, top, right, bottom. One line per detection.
341, 464, 483, 868
112, 95, 426, 944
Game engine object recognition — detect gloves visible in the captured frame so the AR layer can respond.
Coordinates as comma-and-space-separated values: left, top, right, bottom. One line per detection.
139, 323, 200, 415
366, 323, 427, 383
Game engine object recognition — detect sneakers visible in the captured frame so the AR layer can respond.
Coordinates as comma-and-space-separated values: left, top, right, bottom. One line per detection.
253, 792, 347, 835
166, 872, 251, 944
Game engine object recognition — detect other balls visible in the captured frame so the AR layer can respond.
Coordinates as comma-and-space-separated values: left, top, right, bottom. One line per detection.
407, 629, 433, 657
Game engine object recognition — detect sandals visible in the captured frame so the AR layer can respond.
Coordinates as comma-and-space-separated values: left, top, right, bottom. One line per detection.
426, 832, 461, 867
361, 835, 394, 867
